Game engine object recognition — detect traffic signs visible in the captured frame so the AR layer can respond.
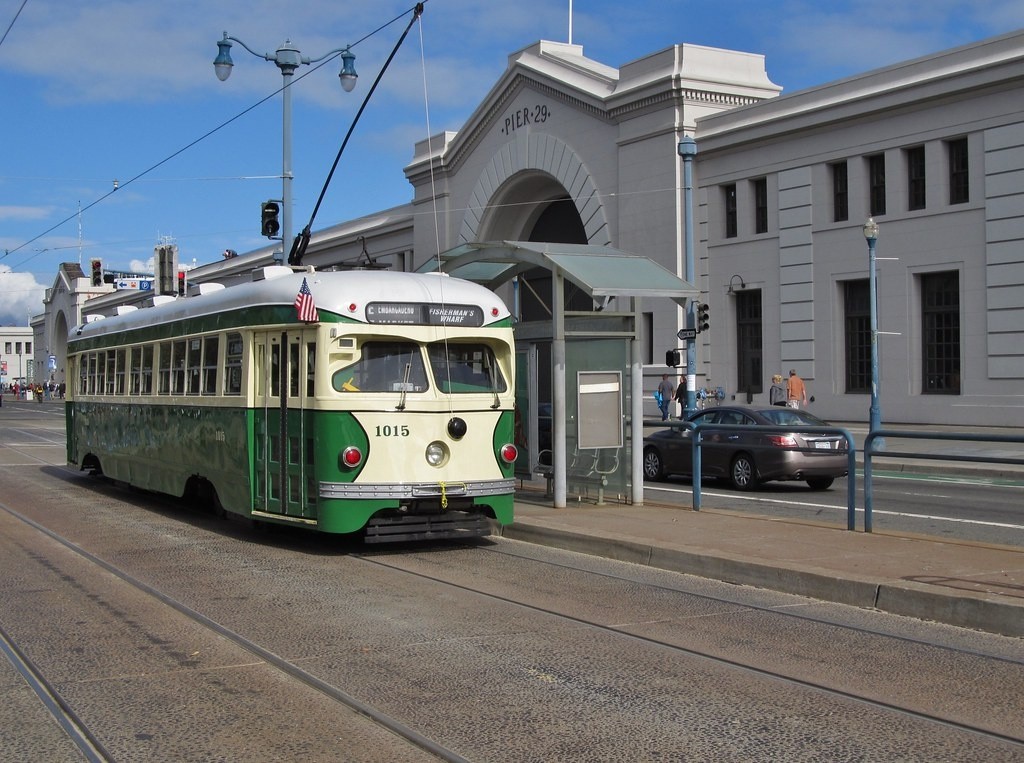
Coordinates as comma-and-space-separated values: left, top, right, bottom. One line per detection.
677, 328, 697, 340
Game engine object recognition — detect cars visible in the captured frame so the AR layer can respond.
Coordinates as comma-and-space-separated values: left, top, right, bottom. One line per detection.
644, 404, 851, 492
538, 402, 553, 467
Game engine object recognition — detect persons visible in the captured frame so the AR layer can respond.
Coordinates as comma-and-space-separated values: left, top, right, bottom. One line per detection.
769, 374, 788, 424
674, 375, 687, 421
786, 369, 808, 410
2, 380, 66, 402
658, 373, 675, 421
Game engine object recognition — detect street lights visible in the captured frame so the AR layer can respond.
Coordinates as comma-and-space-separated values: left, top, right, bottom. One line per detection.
19, 350, 23, 399
45, 346, 50, 400
213, 30, 359, 266
863, 217, 886, 455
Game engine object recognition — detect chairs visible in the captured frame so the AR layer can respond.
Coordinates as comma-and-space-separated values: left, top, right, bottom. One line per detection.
533, 442, 620, 506
720, 415, 737, 424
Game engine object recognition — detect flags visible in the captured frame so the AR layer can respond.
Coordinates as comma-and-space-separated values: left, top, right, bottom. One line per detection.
293, 278, 320, 320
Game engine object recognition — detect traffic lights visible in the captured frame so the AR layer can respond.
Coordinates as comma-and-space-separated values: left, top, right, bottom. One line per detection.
261, 201, 280, 238
697, 302, 710, 332
178, 269, 187, 297
90, 260, 103, 287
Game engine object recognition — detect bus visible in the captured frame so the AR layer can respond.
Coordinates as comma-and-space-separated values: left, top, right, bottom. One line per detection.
62, 263, 518, 551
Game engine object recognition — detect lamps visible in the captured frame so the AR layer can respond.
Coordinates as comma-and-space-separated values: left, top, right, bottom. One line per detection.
726, 274, 745, 296
